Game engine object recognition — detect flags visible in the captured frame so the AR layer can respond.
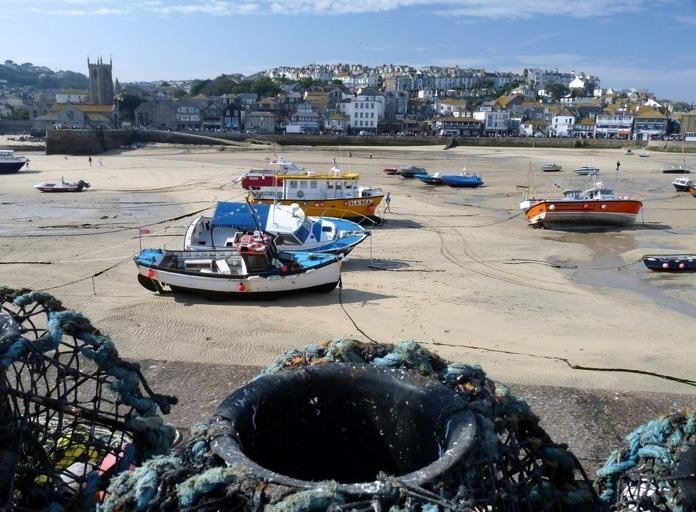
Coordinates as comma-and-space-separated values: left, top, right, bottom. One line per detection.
138, 227, 150, 237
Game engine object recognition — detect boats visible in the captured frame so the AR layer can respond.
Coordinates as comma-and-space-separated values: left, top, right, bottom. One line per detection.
131, 155, 384, 301
518, 140, 643, 228
539, 161, 562, 172
34, 178, 91, 192
383, 165, 484, 189
638, 151, 649, 157
660, 164, 691, 173
0, 149, 30, 174
671, 177, 696, 197
643, 253, 696, 271
625, 148, 634, 155
571, 166, 599, 175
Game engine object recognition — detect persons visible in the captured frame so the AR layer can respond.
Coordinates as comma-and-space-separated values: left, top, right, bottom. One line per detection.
616, 161, 621, 171
383, 191, 392, 213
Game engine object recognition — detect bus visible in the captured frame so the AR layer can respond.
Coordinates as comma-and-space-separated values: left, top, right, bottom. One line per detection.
439, 129, 460, 136
685, 132, 696, 141
